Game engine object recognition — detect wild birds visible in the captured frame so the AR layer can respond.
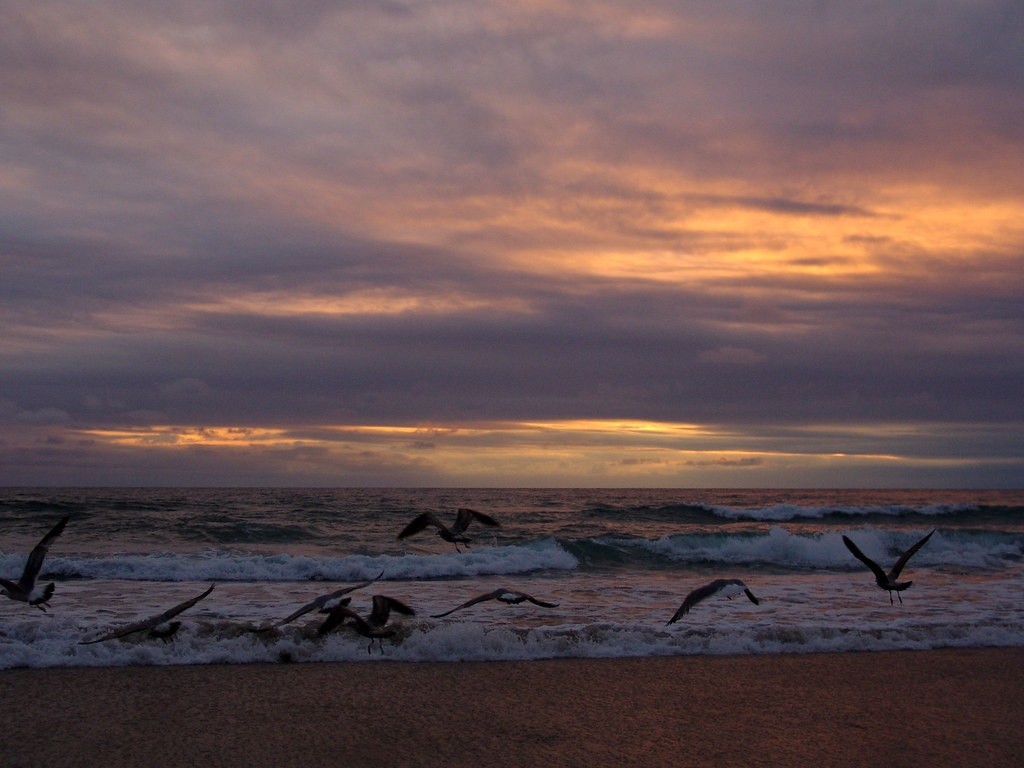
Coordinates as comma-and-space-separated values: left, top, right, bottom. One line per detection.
249, 569, 416, 655
430, 587, 560, 620
77, 581, 216, 645
841, 529, 937, 607
664, 578, 761, 628
0, 515, 72, 614
396, 508, 502, 554
